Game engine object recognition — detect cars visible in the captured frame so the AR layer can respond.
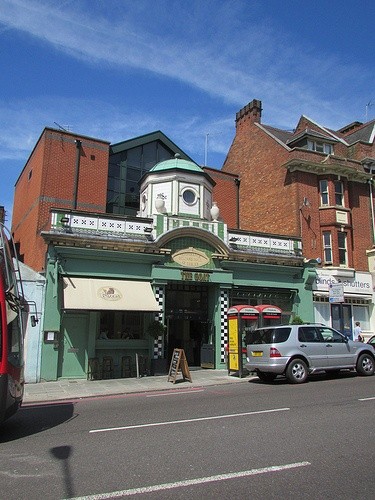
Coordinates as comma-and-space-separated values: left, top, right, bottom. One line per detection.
367, 333, 375, 350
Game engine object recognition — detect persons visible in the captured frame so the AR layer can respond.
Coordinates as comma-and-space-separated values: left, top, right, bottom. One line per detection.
354, 322, 364, 342
120, 328, 134, 340
98, 328, 109, 340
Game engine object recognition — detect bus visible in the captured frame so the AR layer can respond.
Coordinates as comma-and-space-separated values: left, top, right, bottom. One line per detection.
0, 222, 39, 422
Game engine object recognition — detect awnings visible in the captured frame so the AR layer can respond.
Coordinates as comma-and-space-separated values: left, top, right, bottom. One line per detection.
59, 273, 163, 312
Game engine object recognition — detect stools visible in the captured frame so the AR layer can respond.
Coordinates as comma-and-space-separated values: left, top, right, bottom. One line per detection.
87, 357, 100, 381
102, 357, 114, 380
120, 356, 132, 378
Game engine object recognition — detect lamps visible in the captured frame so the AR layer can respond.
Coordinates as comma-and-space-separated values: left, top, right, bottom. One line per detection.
229, 237, 236, 241
60, 217, 69, 223
144, 228, 153, 233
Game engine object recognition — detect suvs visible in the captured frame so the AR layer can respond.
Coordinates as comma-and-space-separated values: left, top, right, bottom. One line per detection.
246, 323, 375, 383
